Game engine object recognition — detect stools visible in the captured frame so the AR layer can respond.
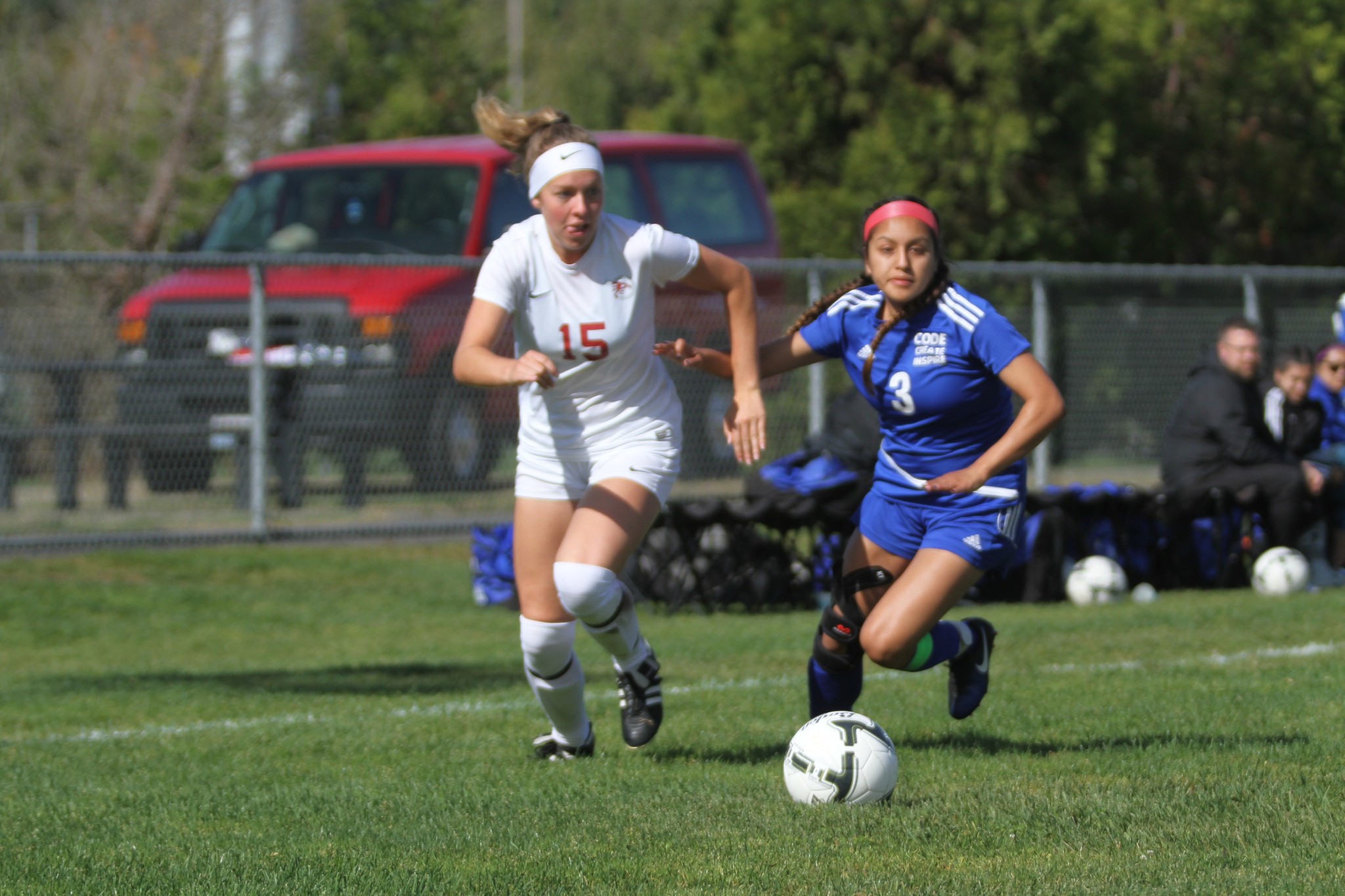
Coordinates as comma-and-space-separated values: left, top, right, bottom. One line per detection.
642, 478, 1269, 612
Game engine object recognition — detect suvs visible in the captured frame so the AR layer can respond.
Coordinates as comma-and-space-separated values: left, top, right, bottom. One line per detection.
117, 129, 791, 494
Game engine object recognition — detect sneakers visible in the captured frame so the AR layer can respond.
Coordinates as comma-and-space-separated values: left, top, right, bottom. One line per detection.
947, 616, 996, 720
533, 723, 597, 764
610, 652, 662, 746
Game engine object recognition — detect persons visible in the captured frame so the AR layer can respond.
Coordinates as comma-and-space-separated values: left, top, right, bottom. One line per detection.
650, 194, 1065, 723
1160, 319, 1344, 590
452, 93, 765, 759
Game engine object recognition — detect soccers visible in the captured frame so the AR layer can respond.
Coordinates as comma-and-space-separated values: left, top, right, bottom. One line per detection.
783, 709, 898, 808
1064, 555, 1128, 605
1252, 546, 1309, 598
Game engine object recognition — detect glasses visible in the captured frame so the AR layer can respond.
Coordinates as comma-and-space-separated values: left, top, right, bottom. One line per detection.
1320, 360, 1345, 372
